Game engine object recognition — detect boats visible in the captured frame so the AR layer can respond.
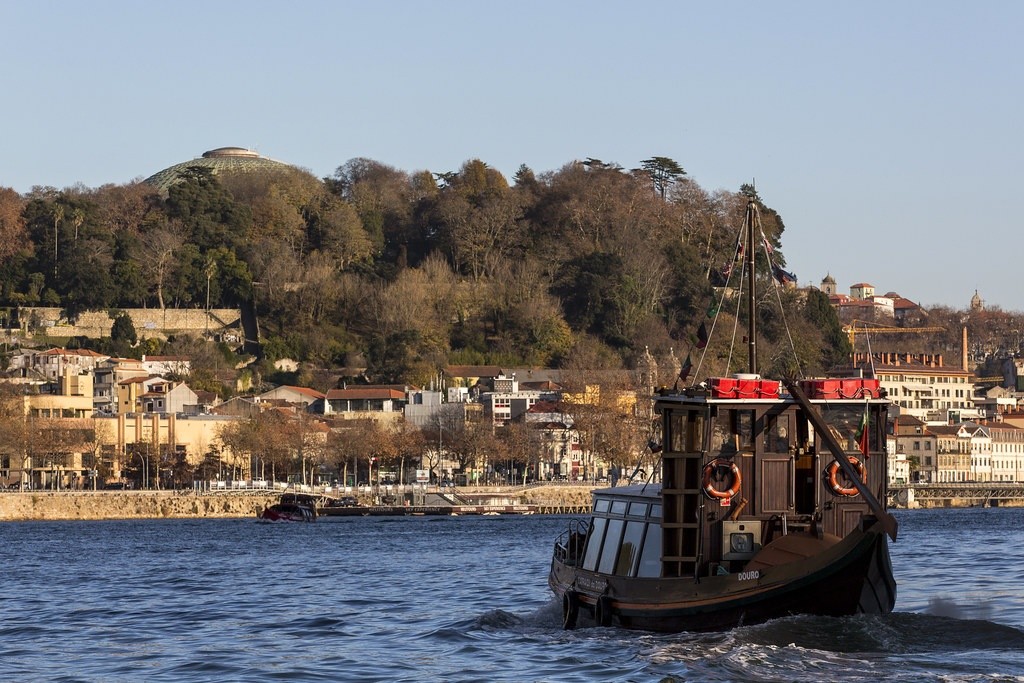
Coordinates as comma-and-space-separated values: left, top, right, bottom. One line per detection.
262, 493, 324, 523
316, 504, 370, 517
548, 179, 899, 634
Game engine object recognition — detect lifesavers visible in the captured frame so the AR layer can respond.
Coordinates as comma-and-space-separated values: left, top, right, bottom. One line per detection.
700, 457, 743, 499
826, 455, 868, 497
